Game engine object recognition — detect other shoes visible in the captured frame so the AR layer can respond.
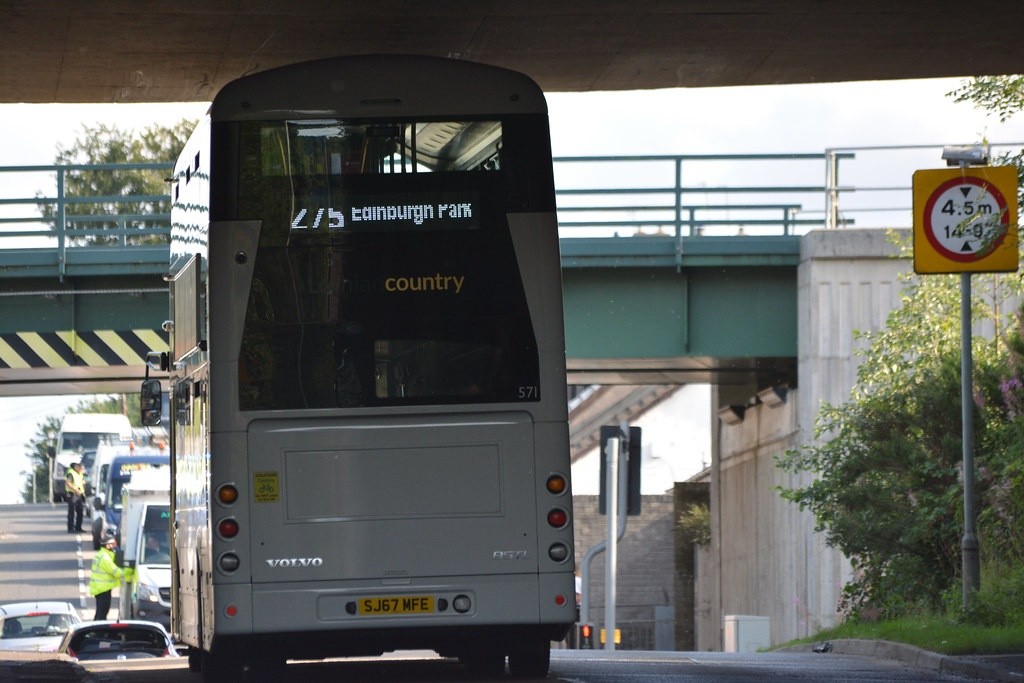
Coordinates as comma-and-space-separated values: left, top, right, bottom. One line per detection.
77, 527, 86, 532
68, 528, 79, 534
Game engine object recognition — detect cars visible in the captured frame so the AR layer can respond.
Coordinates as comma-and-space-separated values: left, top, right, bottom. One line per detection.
57, 619, 182, 661
0, 601, 88, 652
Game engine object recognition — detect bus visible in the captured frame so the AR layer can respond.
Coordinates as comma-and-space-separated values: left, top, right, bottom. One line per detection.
138, 50, 580, 683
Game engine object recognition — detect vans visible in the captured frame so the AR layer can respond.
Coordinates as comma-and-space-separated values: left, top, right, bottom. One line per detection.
77, 441, 172, 626
49, 411, 136, 505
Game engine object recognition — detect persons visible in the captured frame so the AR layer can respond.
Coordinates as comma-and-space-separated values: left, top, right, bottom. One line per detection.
66, 463, 86, 532
144, 535, 170, 564
89, 536, 133, 620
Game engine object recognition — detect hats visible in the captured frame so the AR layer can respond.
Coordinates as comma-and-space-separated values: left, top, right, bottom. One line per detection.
70, 459, 81, 468
100, 537, 115, 544
